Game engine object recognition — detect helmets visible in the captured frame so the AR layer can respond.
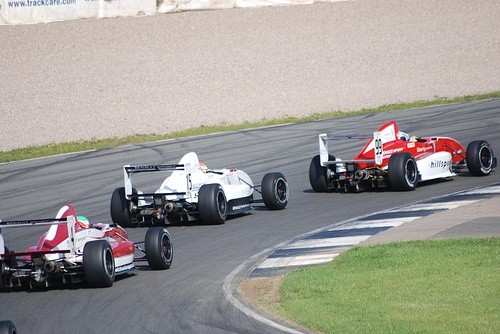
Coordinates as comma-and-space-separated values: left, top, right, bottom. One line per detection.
76, 215, 90, 229
199, 163, 208, 173
397, 131, 410, 142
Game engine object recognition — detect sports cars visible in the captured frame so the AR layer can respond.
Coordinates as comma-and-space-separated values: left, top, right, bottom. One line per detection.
108, 151, 290, 228
308, 120, 497, 192
1, 204, 173, 292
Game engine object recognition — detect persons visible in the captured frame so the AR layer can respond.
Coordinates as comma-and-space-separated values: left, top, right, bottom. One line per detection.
396, 131, 420, 142
199, 163, 209, 174
74, 215, 102, 231
